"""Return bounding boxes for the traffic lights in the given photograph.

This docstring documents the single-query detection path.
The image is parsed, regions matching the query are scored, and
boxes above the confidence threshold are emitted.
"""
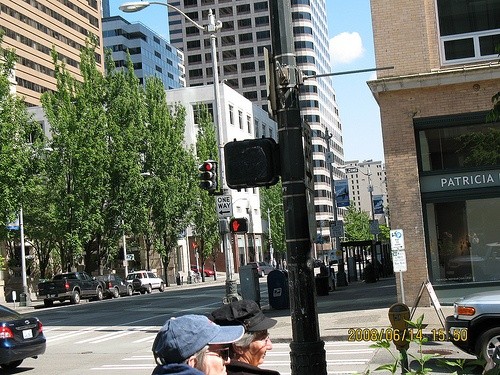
[205,161,218,191]
[199,165,205,189]
[230,218,248,234]
[223,136,279,195]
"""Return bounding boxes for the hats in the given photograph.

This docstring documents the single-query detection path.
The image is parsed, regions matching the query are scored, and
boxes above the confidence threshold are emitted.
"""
[208,299,277,331]
[152,315,244,364]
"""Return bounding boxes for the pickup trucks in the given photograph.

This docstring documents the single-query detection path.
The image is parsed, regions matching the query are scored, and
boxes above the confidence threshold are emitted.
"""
[38,270,104,307]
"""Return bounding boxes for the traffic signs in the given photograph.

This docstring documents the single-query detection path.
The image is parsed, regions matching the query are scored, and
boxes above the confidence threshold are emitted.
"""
[216,195,233,218]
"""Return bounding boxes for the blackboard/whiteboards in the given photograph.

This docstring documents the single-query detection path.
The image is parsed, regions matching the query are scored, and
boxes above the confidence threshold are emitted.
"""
[412,280,442,311]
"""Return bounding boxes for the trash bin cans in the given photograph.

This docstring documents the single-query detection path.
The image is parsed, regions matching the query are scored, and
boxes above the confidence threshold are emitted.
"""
[176,271,183,285]
[187,270,194,284]
[315,274,331,296]
[267,270,290,309]
[363,263,377,283]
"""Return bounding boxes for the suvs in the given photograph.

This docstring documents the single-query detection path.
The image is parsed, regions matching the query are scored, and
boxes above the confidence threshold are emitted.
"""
[248,261,274,277]
[126,270,165,294]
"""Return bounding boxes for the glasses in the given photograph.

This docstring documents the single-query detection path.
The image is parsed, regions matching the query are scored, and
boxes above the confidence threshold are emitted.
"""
[206,343,229,361]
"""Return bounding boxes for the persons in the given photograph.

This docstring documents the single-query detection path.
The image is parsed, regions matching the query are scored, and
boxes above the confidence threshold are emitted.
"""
[151,314,245,375]
[209,299,280,375]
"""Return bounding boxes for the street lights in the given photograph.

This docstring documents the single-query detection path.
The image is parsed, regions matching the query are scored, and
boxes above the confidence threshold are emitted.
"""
[337,165,378,242]
[118,1,244,307]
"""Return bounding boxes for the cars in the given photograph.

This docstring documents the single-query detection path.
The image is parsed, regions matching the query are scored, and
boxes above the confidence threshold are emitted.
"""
[445,289,500,370]
[0,303,47,371]
[191,265,215,277]
[93,274,134,298]
